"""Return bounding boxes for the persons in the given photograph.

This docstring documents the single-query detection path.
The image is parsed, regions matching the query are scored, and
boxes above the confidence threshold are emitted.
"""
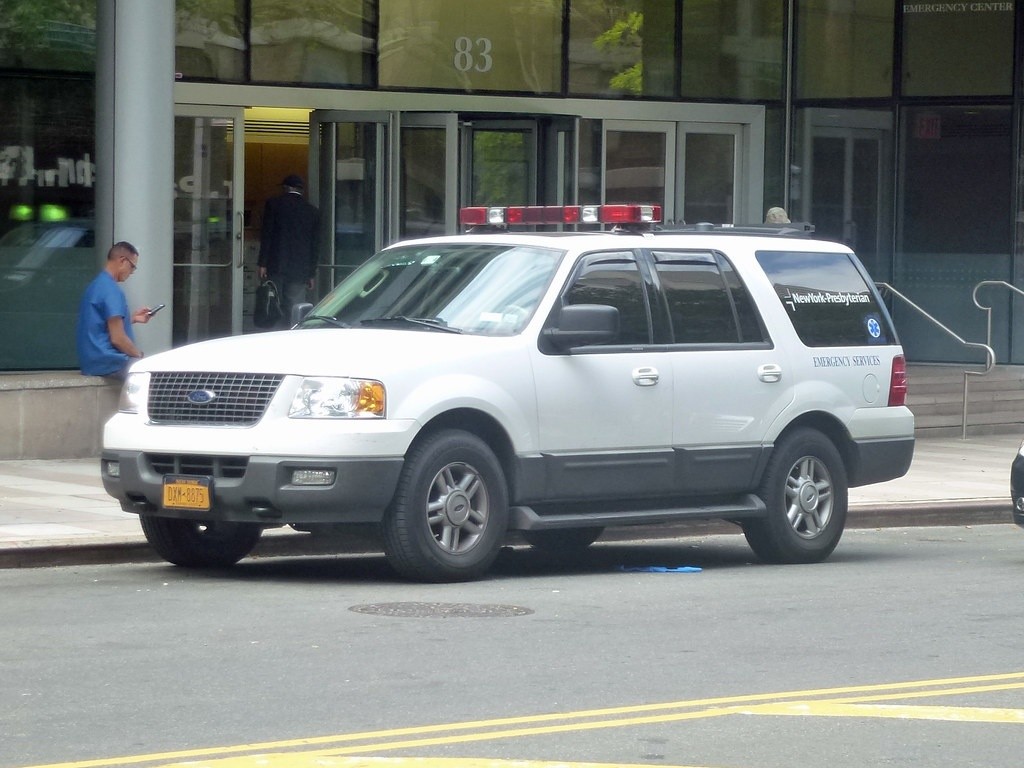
[257,173,325,334]
[76,241,154,380]
[763,206,790,226]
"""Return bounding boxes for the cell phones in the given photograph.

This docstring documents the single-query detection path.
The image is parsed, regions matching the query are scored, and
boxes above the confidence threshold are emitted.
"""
[147,304,165,315]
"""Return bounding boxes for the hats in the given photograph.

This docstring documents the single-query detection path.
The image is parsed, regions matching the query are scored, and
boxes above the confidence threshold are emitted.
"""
[276,174,305,189]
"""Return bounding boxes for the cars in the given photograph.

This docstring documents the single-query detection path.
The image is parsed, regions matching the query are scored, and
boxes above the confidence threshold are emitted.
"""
[2,220,229,368]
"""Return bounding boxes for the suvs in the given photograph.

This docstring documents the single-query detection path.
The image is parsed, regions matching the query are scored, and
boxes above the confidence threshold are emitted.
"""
[100,202,917,585]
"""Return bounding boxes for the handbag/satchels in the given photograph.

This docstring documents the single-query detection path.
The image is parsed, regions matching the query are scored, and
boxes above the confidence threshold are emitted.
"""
[253,271,286,328]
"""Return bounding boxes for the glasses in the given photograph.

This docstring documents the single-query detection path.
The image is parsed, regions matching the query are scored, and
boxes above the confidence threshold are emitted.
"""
[120,255,137,270]
